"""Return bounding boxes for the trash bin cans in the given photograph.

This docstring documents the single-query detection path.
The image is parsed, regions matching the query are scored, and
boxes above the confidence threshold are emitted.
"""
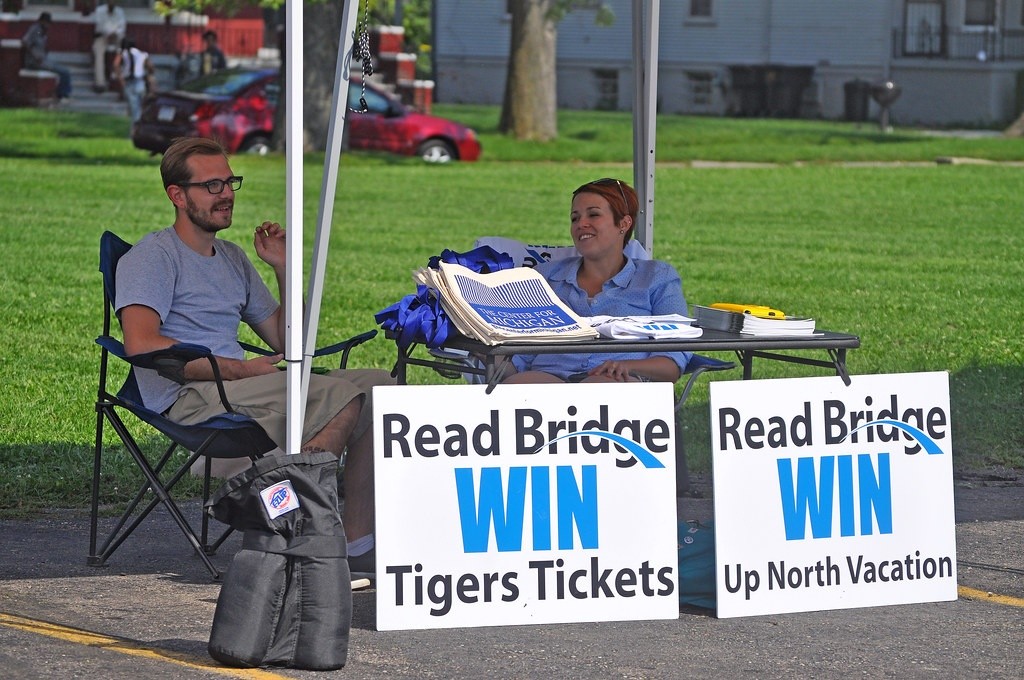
[728,63,814,118]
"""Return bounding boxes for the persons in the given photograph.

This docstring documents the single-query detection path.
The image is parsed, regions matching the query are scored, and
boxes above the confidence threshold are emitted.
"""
[113,135,399,579]
[138,56,160,108]
[21,11,55,106]
[472,177,694,384]
[197,30,227,78]
[112,37,154,140]
[91,0,127,95]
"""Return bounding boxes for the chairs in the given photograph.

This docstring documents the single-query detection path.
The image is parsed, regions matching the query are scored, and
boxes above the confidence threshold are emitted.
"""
[424,236,737,499]
[84,229,378,580]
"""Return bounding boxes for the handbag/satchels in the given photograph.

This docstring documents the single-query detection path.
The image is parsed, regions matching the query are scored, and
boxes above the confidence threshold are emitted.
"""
[372,239,601,355]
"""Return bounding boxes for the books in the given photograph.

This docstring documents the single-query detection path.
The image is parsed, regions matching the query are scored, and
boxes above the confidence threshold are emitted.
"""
[690,303,825,337]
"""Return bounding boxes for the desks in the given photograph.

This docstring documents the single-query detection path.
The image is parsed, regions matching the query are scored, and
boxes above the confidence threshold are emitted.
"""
[382,320,861,387]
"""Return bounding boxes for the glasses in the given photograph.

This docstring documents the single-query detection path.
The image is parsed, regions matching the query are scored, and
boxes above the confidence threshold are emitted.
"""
[572,177,630,215]
[174,175,243,194]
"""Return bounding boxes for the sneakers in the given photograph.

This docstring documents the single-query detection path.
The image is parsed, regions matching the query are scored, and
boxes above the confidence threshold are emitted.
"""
[347,547,376,580]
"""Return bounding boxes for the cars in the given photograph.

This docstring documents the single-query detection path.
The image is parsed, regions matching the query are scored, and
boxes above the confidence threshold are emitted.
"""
[133,68,483,168]
